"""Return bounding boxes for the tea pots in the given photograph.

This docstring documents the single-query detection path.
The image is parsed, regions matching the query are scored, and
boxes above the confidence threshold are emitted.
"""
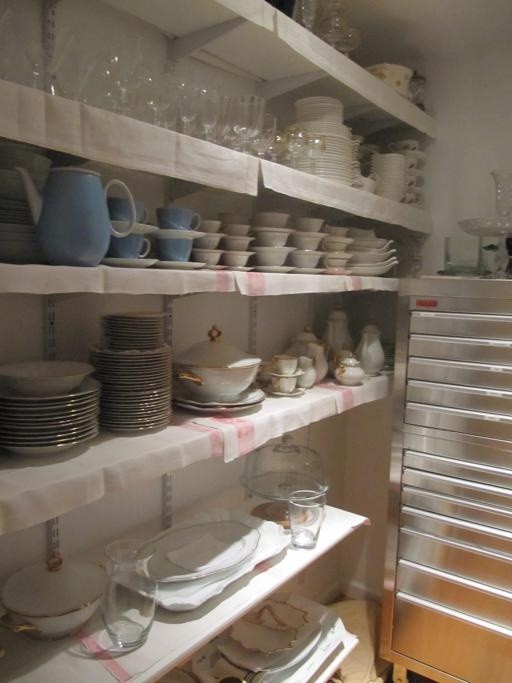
[12,160,138,270]
[294,304,388,388]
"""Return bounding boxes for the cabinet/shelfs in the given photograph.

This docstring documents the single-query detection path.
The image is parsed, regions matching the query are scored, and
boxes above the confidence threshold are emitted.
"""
[0,0,437,683]
[373,272,511,683]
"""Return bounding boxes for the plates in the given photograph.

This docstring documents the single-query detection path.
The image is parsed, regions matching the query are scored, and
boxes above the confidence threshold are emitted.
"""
[175,387,265,414]
[1,162,40,263]
[189,594,346,683]
[130,521,258,586]
[90,309,175,437]
[343,225,401,276]
[0,380,106,459]
[279,94,407,202]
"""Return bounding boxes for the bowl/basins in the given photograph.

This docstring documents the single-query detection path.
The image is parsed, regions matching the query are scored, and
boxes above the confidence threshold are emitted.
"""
[1,357,96,396]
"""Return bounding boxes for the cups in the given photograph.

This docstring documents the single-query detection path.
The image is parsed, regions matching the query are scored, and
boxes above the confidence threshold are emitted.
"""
[269,352,304,397]
[364,61,414,100]
[490,168,511,216]
[197,208,354,274]
[389,136,425,206]
[100,195,206,267]
[286,489,329,555]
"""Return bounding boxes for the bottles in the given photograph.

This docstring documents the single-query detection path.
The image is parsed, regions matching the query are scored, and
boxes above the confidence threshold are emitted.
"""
[101,536,156,651]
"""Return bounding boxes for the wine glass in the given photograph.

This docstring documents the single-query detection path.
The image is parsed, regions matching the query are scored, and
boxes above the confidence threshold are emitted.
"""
[11,14,329,173]
[455,215,512,280]
[298,0,361,58]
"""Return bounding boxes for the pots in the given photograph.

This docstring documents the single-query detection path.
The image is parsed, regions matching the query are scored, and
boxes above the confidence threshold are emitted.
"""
[2,553,107,643]
[174,323,262,402]
